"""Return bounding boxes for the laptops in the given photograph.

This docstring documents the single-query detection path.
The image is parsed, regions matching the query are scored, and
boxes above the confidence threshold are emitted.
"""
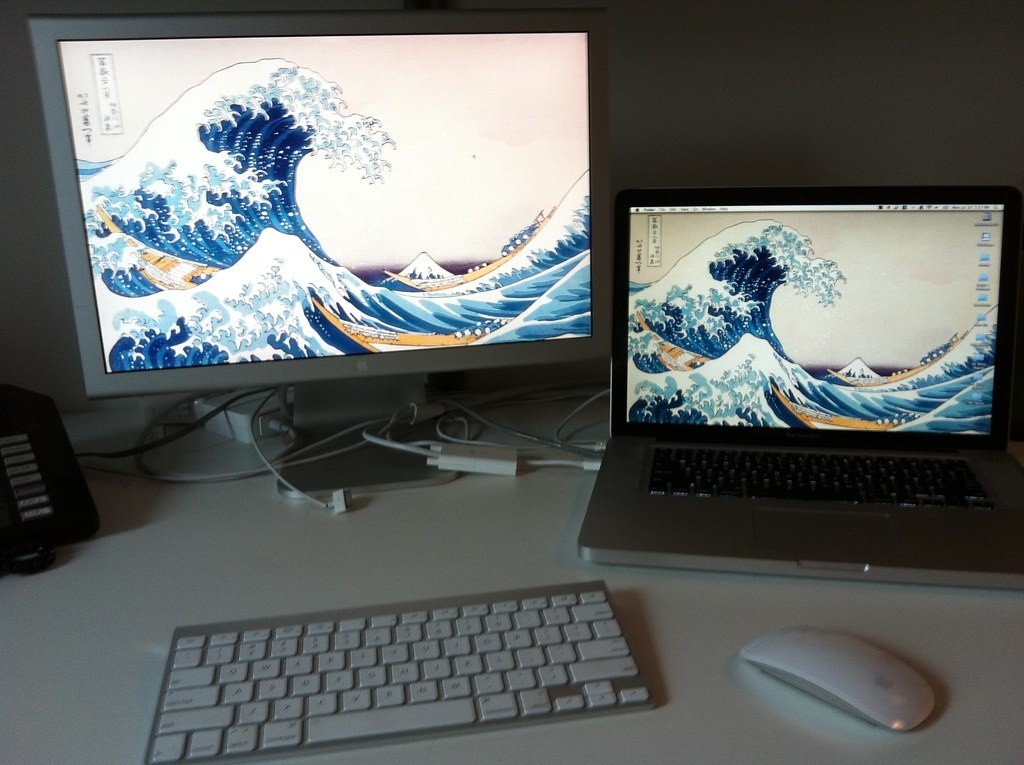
[577,187,1023,589]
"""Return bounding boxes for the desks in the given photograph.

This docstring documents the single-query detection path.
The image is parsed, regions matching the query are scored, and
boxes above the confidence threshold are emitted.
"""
[0,383,1021,762]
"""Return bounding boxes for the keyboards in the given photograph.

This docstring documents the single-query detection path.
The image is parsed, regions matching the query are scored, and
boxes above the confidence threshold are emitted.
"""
[144,580,656,765]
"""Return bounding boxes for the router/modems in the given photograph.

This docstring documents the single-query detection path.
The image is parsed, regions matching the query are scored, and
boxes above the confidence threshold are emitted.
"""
[192,391,295,443]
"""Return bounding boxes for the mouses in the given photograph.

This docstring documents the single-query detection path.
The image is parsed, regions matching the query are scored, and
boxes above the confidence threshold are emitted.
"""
[741,625,938,732]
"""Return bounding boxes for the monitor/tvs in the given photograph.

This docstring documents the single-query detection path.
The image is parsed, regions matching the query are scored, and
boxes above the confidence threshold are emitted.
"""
[23,13,613,497]
